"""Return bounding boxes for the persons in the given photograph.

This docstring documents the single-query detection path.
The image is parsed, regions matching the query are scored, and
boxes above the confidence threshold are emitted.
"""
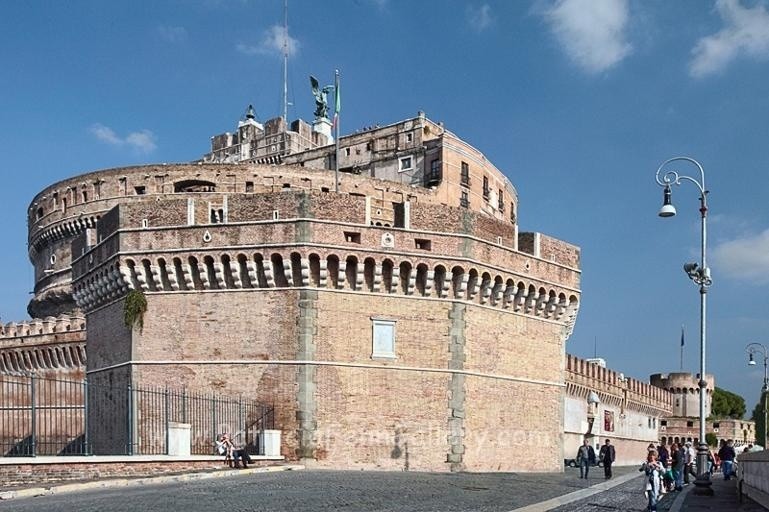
[599,438,616,479]
[576,439,595,479]
[222,433,256,468]
[320,87,328,118]
[639,438,754,511]
[215,433,244,468]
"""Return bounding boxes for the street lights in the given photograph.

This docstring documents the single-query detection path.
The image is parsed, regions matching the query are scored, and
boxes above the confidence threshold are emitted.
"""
[744,342,768,450]
[654,155,715,496]
